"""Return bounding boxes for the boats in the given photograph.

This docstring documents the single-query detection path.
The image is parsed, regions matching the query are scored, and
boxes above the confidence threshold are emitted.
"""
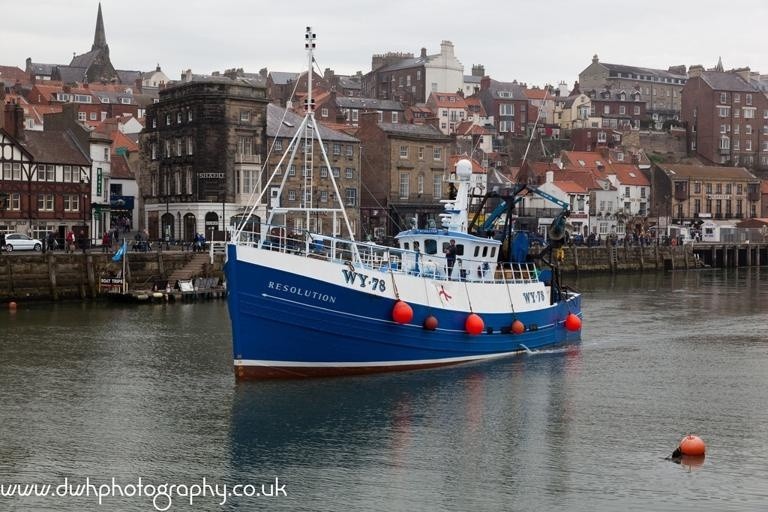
[224,21,593,382]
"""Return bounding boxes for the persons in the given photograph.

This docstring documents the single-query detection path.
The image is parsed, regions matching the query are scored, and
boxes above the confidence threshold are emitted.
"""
[194,233,201,252]
[46,217,151,253]
[200,234,206,251]
[694,231,699,243]
[443,239,456,281]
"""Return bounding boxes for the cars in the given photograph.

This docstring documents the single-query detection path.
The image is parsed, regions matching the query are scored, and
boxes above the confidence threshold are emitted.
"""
[2,233,48,253]
